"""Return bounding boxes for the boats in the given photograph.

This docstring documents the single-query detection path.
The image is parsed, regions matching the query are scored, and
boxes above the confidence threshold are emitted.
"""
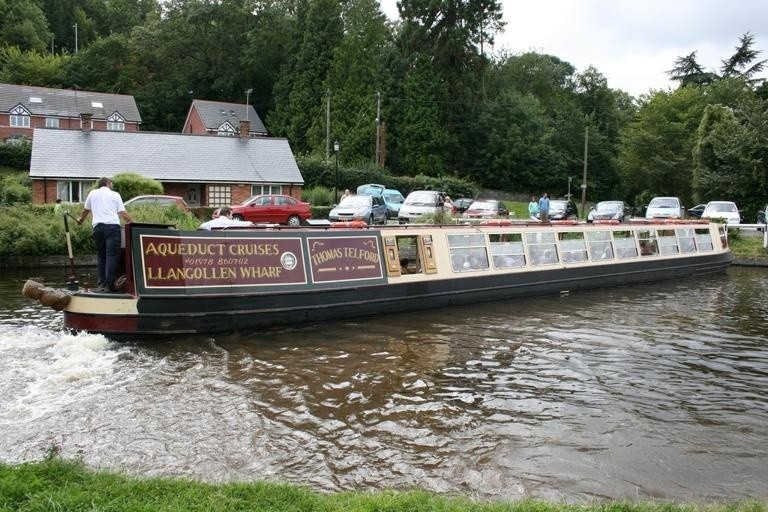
[50,202,735,340]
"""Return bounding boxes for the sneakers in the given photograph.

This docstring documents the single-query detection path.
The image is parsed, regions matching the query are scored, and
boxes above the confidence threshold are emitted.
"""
[104,283,119,293]
[98,282,105,291]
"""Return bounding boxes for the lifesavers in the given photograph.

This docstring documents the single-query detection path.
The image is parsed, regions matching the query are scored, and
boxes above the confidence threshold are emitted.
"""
[550,218,581,226]
[480,218,511,227]
[594,219,620,226]
[331,220,367,231]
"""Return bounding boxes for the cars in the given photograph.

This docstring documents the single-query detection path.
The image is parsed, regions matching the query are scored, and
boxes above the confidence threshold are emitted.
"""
[686,204,707,219]
[586,201,626,223]
[399,190,447,224]
[209,190,313,228]
[324,183,391,228]
[462,200,509,222]
[756,206,767,230]
[452,197,474,213]
[701,202,741,230]
[645,197,685,221]
[379,189,407,216]
[536,201,581,224]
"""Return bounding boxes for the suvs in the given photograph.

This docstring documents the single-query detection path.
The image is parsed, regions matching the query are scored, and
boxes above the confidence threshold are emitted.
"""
[118,195,197,223]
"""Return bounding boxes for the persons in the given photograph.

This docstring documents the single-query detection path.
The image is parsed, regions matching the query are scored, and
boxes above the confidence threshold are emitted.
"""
[444,195,456,218]
[400,258,411,274]
[452,241,654,270]
[537,191,550,221]
[340,189,351,202]
[529,195,539,220]
[52,196,64,216]
[198,205,255,231]
[77,177,137,293]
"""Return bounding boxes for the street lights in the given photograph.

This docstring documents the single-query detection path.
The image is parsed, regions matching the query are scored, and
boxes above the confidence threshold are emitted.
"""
[333,137,340,204]
